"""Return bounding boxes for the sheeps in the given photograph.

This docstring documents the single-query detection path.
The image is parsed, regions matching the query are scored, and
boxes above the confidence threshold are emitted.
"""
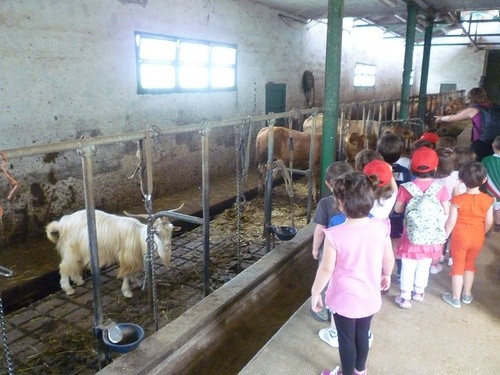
[45,202,184,299]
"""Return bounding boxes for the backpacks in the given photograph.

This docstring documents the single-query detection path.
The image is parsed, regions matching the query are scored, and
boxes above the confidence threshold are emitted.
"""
[399,180,447,245]
[471,104,500,144]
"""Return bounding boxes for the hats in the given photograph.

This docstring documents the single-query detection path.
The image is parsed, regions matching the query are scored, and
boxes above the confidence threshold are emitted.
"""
[412,146,439,172]
[420,131,441,143]
[363,160,392,187]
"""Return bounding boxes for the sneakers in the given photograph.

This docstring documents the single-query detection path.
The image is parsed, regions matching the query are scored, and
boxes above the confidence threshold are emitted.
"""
[321,366,342,375]
[461,293,473,304]
[368,334,374,348]
[318,327,339,347]
[354,366,367,375]
[395,295,411,309]
[442,293,461,308]
[412,290,423,301]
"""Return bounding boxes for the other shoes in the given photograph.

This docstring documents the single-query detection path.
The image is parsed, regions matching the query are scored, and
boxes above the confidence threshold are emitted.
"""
[396,273,401,281]
[430,264,442,273]
[439,255,445,262]
[311,305,330,321]
[447,257,453,266]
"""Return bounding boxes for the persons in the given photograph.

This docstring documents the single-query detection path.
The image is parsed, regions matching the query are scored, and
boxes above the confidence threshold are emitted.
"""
[434,87,500,142]
[394,146,450,309]
[354,128,496,295]
[442,161,494,308]
[310,161,355,322]
[311,171,395,375]
[481,135,500,226]
[319,213,375,348]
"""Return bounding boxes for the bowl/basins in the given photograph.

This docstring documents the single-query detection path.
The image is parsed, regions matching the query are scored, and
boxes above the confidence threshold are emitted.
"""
[275,227,297,240]
[101,322,144,353]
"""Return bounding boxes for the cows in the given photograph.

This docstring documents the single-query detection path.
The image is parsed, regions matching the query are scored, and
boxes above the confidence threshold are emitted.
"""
[255,94,467,205]
